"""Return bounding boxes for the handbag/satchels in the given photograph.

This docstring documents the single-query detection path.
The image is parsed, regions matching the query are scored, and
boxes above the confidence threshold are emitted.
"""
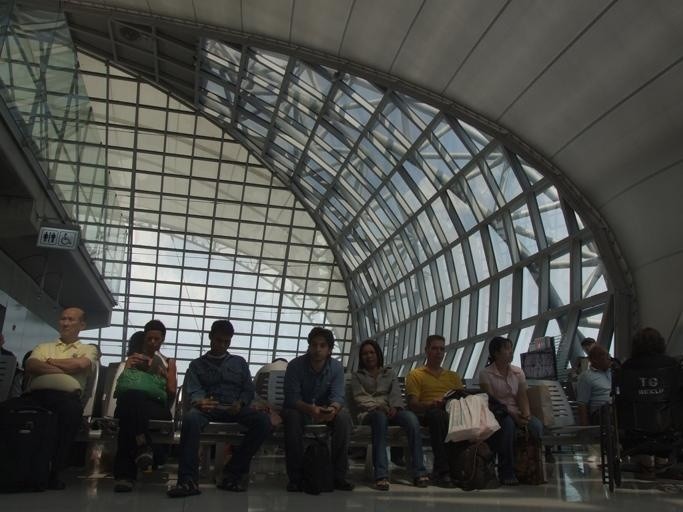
[452,440,499,492]
[513,426,542,486]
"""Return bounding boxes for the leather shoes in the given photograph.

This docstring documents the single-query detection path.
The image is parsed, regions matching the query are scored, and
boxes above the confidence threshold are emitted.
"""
[50,473,65,490]
[286,479,303,492]
[390,456,407,467]
[335,477,355,490]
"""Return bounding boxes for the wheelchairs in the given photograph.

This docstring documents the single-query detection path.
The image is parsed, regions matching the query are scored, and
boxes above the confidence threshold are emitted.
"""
[600,353,683,493]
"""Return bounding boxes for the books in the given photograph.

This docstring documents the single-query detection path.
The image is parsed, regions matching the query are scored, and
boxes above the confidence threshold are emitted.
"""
[146,350,169,376]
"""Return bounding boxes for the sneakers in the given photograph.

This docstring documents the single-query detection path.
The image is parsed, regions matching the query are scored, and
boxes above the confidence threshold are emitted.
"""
[134,446,153,468]
[113,475,134,491]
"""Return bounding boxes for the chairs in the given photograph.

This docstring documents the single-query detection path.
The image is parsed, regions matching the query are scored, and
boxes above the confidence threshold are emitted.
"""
[0,354,600,489]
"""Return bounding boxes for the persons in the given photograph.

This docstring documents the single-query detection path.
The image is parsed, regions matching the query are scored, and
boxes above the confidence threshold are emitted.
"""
[403,333,499,487]
[579,335,622,386]
[22,306,99,490]
[112,318,177,493]
[349,338,431,490]
[163,319,272,498]
[279,326,356,491]
[126,330,144,354]
[0,303,7,349]
[613,325,682,473]
[477,335,548,485]
[574,344,669,473]
[86,341,105,416]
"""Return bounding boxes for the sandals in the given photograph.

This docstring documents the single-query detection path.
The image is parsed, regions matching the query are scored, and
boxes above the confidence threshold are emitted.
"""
[376,478,389,491]
[168,482,202,496]
[216,476,244,491]
[414,475,430,488]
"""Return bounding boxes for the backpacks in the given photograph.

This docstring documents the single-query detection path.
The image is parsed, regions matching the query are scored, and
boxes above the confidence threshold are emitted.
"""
[306,431,334,496]
[1,398,58,493]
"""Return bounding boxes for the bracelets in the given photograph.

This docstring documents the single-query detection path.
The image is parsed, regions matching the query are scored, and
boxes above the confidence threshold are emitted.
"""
[329,404,337,411]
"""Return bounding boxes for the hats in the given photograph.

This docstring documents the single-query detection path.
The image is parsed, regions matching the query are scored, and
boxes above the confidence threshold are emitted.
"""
[581,338,594,346]
